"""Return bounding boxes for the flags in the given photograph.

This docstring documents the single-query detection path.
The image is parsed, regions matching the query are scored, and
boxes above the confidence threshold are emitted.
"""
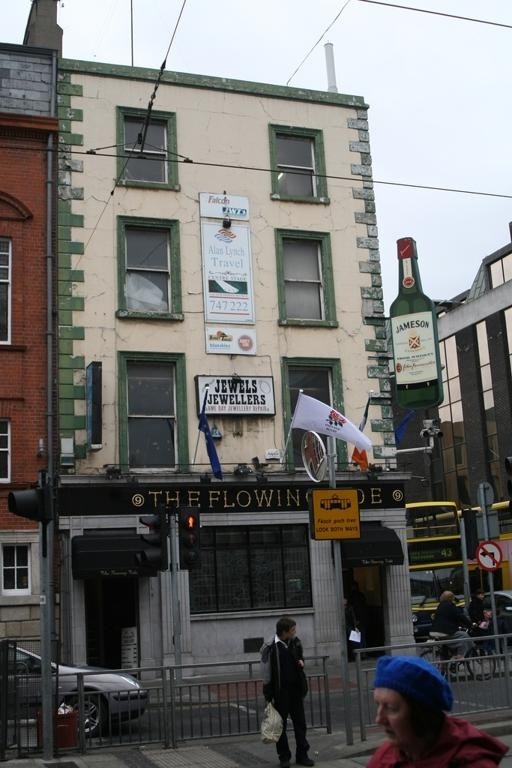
[393,408,414,440]
[197,391,223,480]
[291,393,371,450]
[352,395,371,473]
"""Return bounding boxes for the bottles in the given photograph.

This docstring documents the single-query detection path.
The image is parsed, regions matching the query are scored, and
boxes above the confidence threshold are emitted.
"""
[387,235,444,410]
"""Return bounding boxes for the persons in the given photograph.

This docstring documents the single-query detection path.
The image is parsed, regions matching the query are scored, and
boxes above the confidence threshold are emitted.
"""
[257,618,315,767]
[364,655,509,768]
[465,592,494,655]
[431,588,481,678]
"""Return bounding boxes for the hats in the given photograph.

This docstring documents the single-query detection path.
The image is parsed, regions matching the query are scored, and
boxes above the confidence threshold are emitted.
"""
[375,654,453,717]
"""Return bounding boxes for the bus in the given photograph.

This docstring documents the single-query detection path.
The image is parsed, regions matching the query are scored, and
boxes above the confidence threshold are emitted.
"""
[404,501,511,649]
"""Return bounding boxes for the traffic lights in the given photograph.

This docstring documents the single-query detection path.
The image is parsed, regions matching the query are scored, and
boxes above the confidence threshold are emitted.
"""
[178,505,203,570]
[138,503,169,569]
[503,456,512,507]
[8,483,52,526]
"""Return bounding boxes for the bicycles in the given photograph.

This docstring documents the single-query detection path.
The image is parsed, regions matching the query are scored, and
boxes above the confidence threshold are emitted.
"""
[419,625,496,681]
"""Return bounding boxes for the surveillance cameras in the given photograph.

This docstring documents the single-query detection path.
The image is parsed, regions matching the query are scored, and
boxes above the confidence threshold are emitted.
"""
[419,418,443,440]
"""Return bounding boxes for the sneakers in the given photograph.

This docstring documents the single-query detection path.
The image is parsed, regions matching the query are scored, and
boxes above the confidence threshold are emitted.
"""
[281,760,293,767]
[296,757,314,766]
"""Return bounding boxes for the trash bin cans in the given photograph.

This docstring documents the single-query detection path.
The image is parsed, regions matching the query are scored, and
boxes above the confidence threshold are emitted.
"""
[36,708,79,749]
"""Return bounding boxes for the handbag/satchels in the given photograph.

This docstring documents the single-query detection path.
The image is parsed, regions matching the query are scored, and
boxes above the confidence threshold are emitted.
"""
[260,702,284,744]
[298,666,308,700]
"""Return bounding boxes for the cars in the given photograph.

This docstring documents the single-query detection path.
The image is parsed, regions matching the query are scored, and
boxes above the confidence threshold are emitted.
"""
[1,641,151,741]
[263,568,306,605]
[190,577,242,613]
